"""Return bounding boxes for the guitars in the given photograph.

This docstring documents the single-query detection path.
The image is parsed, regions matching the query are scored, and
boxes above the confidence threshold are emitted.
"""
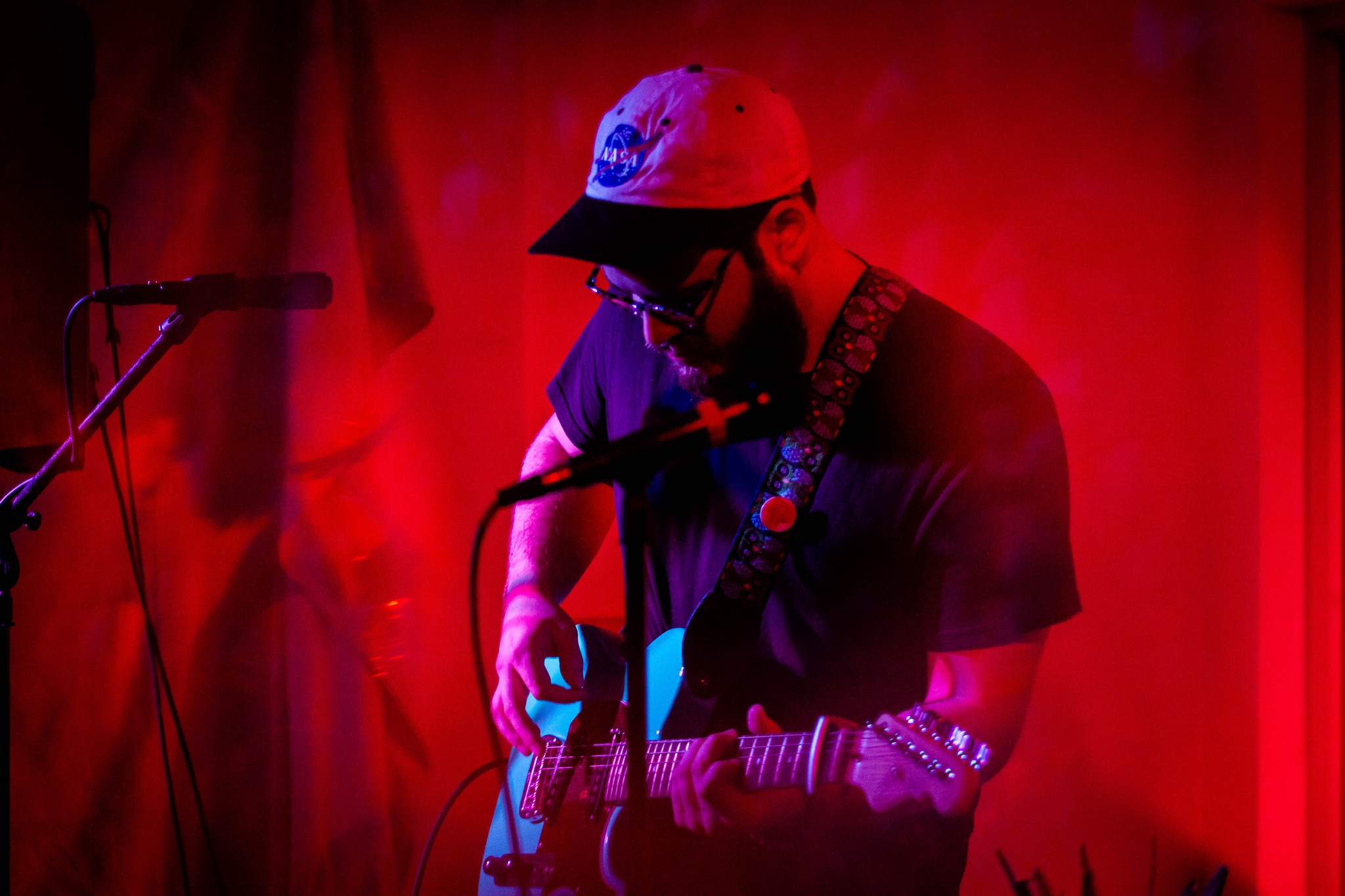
[475,623,1000,895]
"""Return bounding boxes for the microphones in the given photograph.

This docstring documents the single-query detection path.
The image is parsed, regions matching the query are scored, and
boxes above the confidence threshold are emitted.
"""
[497,381,772,511]
[98,270,332,311]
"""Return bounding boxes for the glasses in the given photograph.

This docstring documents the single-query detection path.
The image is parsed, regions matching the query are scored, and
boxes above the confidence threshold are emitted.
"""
[578,242,745,338]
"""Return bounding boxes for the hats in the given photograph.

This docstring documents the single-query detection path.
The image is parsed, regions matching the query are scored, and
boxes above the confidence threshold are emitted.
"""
[527,63,817,290]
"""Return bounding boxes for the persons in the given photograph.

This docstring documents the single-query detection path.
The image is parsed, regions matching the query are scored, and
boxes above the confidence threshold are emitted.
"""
[488,67,1082,896]
